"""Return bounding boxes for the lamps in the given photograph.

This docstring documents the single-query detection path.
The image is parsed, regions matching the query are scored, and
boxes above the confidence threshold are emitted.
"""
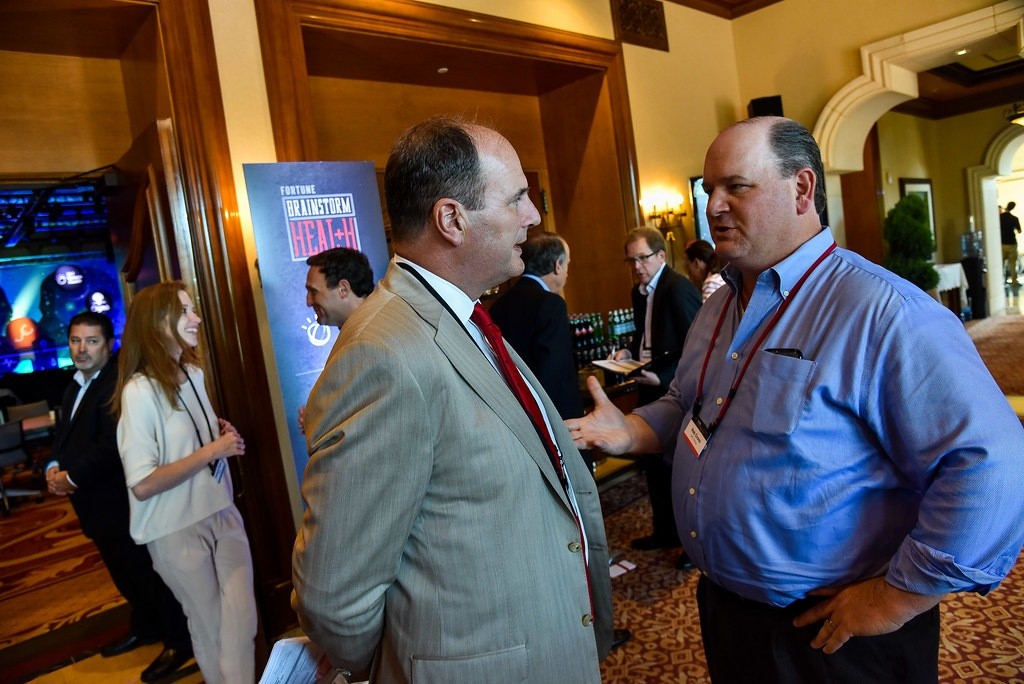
[639,185,689,244]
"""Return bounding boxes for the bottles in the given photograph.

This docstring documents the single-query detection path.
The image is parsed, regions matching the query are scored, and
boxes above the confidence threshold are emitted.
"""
[961,231,984,259]
[570,308,634,372]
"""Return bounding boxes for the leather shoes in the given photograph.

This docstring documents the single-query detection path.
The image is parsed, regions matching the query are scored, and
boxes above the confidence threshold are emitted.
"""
[612,628,631,647]
[100,632,156,657]
[140,638,194,683]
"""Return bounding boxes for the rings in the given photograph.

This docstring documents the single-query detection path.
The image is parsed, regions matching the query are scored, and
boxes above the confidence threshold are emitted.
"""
[828,618,837,632]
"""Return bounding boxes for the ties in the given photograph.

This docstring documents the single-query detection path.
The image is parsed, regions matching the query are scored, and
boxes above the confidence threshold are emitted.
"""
[473,299,566,493]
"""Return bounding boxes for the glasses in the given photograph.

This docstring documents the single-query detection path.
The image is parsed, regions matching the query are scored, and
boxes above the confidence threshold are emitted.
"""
[625,248,660,265]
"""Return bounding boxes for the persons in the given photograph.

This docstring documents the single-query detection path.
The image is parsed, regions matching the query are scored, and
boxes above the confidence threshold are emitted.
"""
[685,240,728,305]
[44,312,195,682]
[485,231,630,649]
[998,202,1024,287]
[99,279,256,684]
[287,112,616,684]
[298,247,375,435]
[563,117,1024,684]
[608,225,702,570]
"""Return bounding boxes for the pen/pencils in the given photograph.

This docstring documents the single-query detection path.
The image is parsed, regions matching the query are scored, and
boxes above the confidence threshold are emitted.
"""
[611,345,616,360]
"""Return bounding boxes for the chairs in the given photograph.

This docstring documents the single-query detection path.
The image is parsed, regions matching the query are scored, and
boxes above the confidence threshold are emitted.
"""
[0,404,75,516]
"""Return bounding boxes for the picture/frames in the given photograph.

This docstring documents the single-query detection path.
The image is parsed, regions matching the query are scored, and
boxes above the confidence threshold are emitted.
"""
[899,176,937,250]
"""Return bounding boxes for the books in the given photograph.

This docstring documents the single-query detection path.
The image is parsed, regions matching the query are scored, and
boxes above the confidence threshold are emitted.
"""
[592,357,651,379]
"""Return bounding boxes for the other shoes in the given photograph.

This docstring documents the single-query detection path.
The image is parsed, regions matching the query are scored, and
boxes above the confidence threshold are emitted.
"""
[675,553,696,571]
[631,531,683,551]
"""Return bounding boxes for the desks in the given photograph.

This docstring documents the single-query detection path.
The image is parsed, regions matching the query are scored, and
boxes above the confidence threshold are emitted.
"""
[24,413,56,432]
[928,263,969,310]
